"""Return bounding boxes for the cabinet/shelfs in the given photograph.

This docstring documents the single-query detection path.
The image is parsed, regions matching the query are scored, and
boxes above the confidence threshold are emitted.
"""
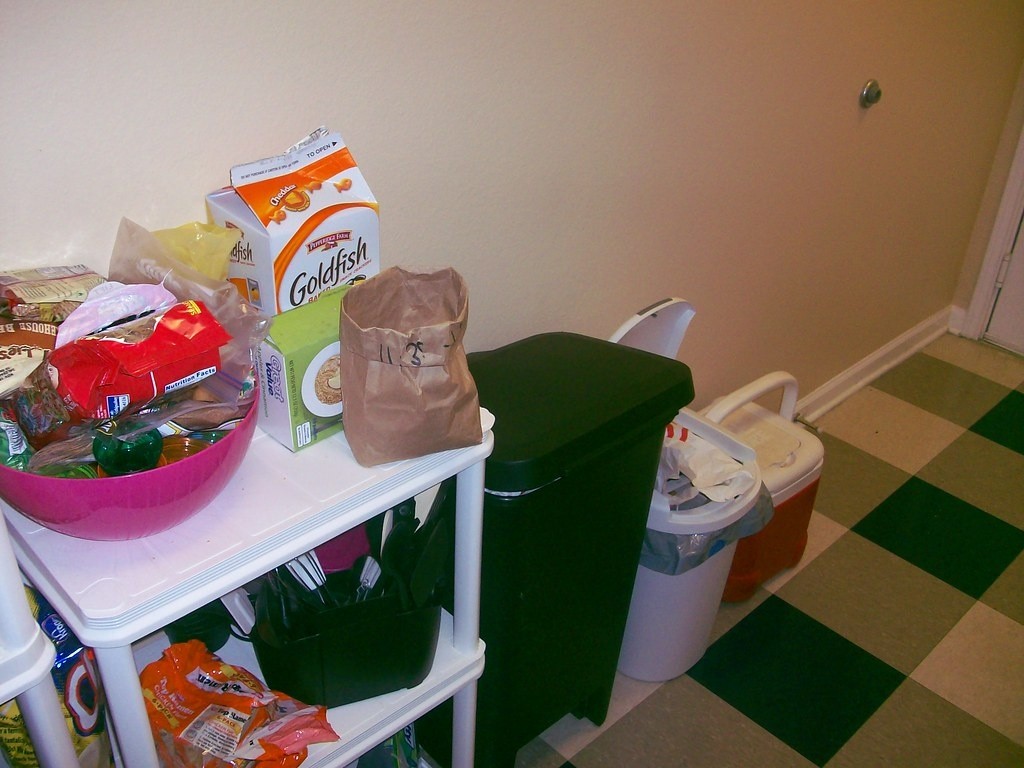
[0,406,494,768]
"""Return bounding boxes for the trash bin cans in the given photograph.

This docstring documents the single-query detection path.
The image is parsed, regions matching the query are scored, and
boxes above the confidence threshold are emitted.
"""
[607,294,765,682]
[410,328,698,766]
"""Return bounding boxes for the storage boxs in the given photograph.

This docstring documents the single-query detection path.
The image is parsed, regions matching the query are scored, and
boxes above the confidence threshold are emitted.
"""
[256,286,353,453]
[697,371,823,602]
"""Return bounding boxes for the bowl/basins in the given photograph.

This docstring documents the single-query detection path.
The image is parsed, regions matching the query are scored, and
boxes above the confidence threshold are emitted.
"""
[0,368,261,542]
